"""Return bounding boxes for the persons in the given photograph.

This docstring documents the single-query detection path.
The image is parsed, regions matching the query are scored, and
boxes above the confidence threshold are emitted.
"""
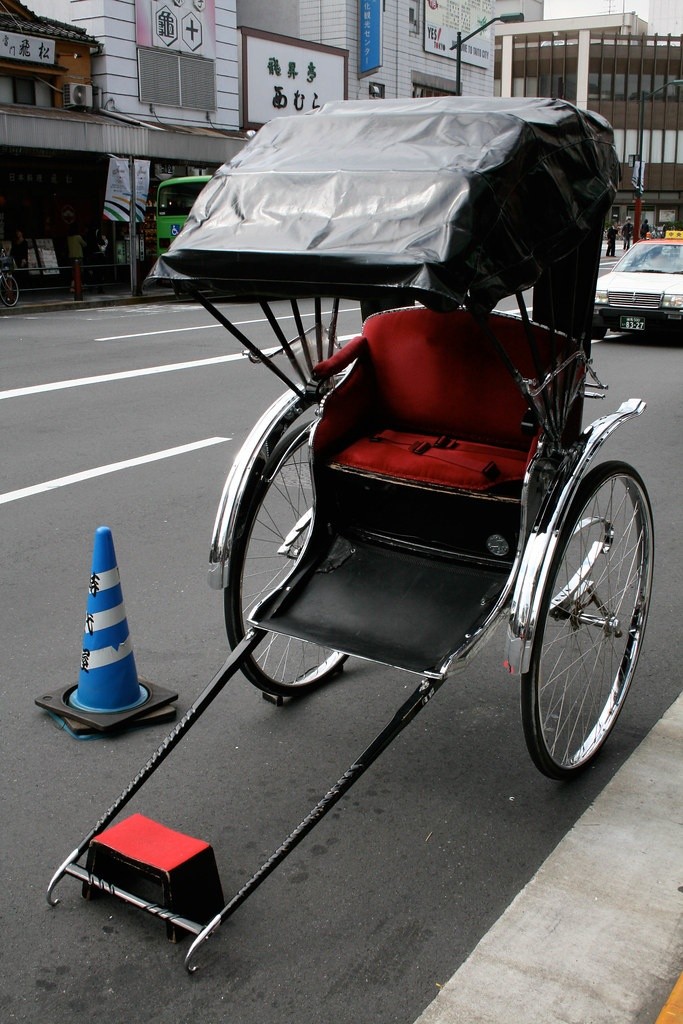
[621,219,633,251]
[606,222,618,257]
[9,231,29,269]
[641,219,649,237]
[66,226,87,291]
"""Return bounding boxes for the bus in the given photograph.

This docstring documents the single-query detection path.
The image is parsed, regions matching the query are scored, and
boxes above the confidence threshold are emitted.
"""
[150,175,215,259]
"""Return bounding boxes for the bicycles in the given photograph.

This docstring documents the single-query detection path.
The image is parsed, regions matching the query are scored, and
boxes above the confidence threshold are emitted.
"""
[0,249,19,307]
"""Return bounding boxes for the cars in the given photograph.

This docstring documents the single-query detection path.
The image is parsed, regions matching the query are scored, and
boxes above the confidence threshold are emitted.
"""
[591,230,683,345]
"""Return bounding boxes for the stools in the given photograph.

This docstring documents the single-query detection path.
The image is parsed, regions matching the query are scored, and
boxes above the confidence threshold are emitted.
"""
[81,813,226,942]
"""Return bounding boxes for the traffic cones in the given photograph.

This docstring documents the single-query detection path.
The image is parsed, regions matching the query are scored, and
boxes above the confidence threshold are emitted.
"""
[35,527,180,739]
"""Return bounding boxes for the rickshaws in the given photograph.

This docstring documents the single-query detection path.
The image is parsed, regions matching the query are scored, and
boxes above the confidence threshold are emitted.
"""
[44,95,658,977]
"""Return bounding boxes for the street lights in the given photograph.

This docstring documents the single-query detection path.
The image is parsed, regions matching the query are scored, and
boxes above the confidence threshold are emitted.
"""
[449,12,524,96]
[633,80,683,245]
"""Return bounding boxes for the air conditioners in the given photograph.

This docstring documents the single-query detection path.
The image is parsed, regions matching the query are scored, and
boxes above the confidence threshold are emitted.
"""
[63,82,93,108]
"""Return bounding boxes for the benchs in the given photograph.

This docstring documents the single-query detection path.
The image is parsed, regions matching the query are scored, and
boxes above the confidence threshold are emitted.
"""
[312,306,586,502]
